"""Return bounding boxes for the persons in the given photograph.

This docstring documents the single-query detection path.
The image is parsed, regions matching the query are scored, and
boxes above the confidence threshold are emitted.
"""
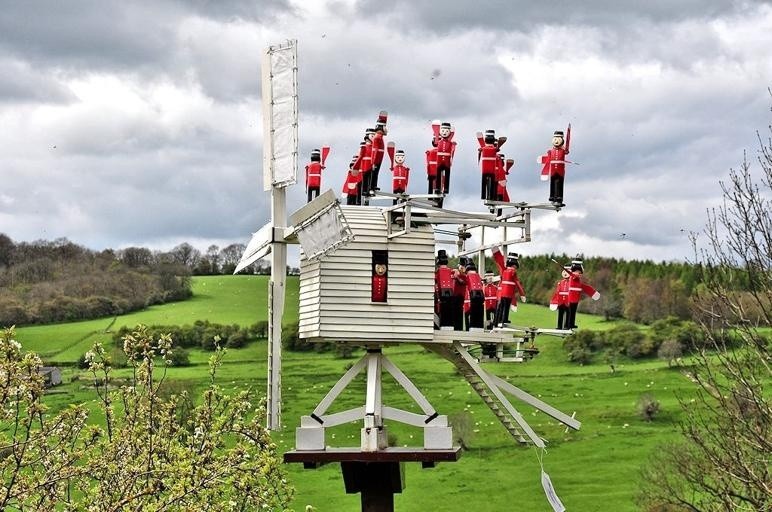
[306,147,330,203]
[434,247,527,331]
[372,259,388,302]
[425,120,457,209]
[476,129,514,222]
[537,123,571,208]
[305,111,599,334]
[343,112,410,207]
[549,257,599,330]
